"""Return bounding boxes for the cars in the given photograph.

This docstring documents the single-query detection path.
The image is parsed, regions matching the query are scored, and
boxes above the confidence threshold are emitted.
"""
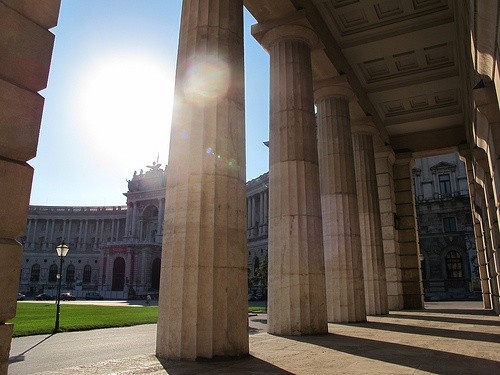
[60,293,76,301]
[85,291,104,300]
[17,293,26,301]
[34,293,55,300]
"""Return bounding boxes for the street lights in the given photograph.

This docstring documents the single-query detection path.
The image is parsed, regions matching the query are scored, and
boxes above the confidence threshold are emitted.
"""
[52,240,71,333]
[55,273,63,303]
